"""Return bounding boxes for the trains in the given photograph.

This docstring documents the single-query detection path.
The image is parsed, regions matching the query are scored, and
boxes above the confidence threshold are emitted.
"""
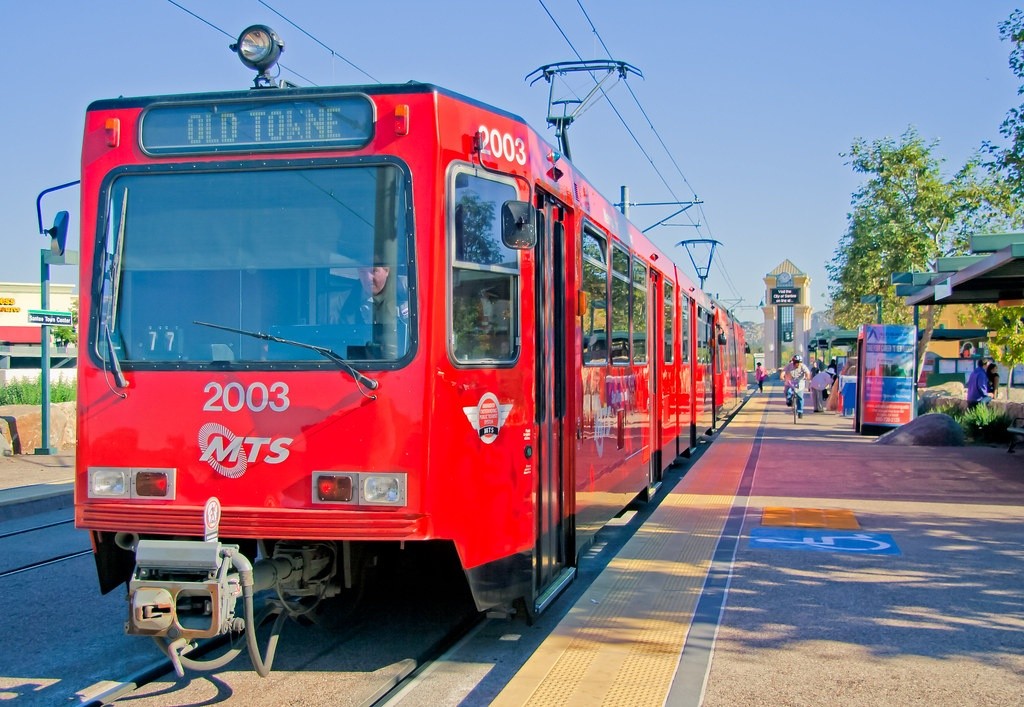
[37,23,750,677]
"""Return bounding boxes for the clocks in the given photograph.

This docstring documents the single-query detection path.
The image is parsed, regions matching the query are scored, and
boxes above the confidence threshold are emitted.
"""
[779,272,791,284]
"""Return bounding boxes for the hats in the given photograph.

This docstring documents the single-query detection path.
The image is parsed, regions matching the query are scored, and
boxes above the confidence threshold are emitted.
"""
[826,368,836,375]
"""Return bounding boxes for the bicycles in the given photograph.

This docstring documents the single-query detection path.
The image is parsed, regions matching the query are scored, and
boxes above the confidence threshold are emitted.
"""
[780,379,807,425]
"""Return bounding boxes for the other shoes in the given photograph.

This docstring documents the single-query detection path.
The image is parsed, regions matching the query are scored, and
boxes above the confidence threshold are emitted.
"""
[798,413,802,418]
[786,400,792,406]
[814,409,824,413]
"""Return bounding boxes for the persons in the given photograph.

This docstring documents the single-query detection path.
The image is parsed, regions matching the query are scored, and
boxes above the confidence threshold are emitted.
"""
[779,355,811,419]
[811,368,836,412]
[341,252,408,361]
[810,362,819,378]
[756,362,767,393]
[966,357,999,410]
[828,359,837,387]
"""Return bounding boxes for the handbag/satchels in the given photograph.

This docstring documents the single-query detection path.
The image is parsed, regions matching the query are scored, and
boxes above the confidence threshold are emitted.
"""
[822,389,829,401]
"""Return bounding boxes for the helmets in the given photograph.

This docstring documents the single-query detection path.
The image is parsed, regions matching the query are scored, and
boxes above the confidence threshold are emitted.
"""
[792,355,802,362]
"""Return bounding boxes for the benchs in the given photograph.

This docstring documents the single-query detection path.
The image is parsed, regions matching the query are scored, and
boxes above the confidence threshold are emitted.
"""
[1007,417,1024,452]
